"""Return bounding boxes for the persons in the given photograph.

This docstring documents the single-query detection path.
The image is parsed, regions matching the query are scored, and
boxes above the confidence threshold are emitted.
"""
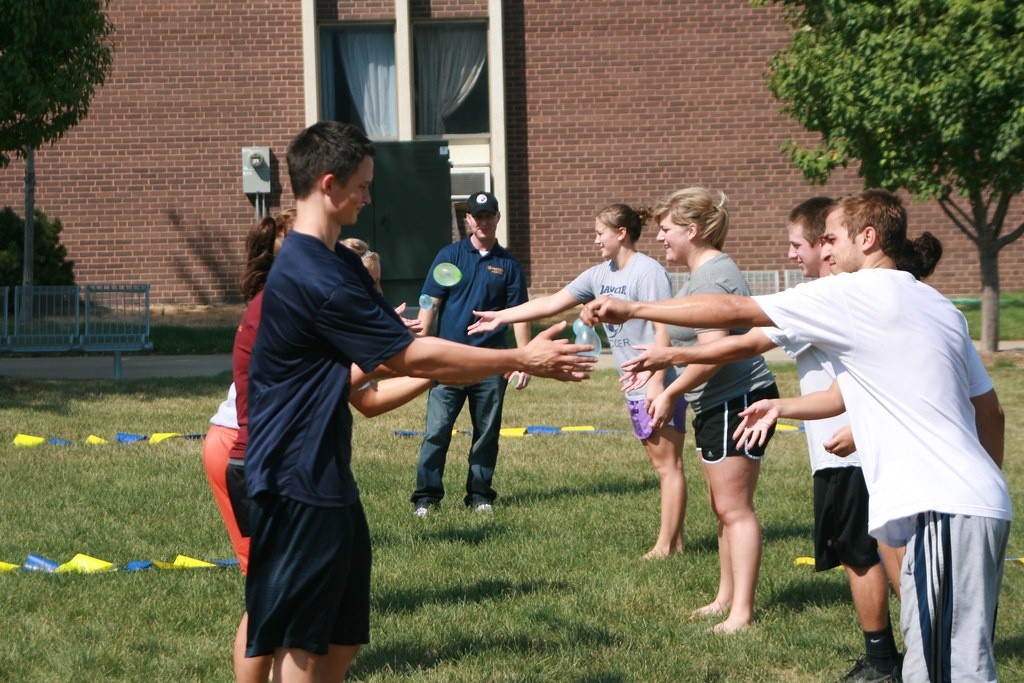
[620,197,907,683]
[242,118,600,683]
[202,237,429,577]
[224,209,298,682]
[617,188,781,634]
[466,202,688,559]
[410,192,532,518]
[581,190,1010,683]
[731,231,943,599]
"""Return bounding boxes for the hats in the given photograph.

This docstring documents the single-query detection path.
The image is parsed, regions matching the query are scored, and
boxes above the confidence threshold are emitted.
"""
[466,190,498,217]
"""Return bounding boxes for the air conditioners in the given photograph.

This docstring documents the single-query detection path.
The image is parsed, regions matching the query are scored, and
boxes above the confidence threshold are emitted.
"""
[436,167,490,201]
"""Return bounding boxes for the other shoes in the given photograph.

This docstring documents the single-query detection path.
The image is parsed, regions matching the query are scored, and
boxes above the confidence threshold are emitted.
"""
[471,502,493,513]
[412,501,428,517]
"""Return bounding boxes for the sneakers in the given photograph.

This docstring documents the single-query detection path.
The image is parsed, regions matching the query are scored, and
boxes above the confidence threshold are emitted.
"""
[836,652,905,683]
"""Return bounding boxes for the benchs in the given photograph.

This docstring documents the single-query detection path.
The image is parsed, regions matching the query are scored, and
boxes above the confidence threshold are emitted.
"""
[1,283,157,378]
[659,268,818,294]
